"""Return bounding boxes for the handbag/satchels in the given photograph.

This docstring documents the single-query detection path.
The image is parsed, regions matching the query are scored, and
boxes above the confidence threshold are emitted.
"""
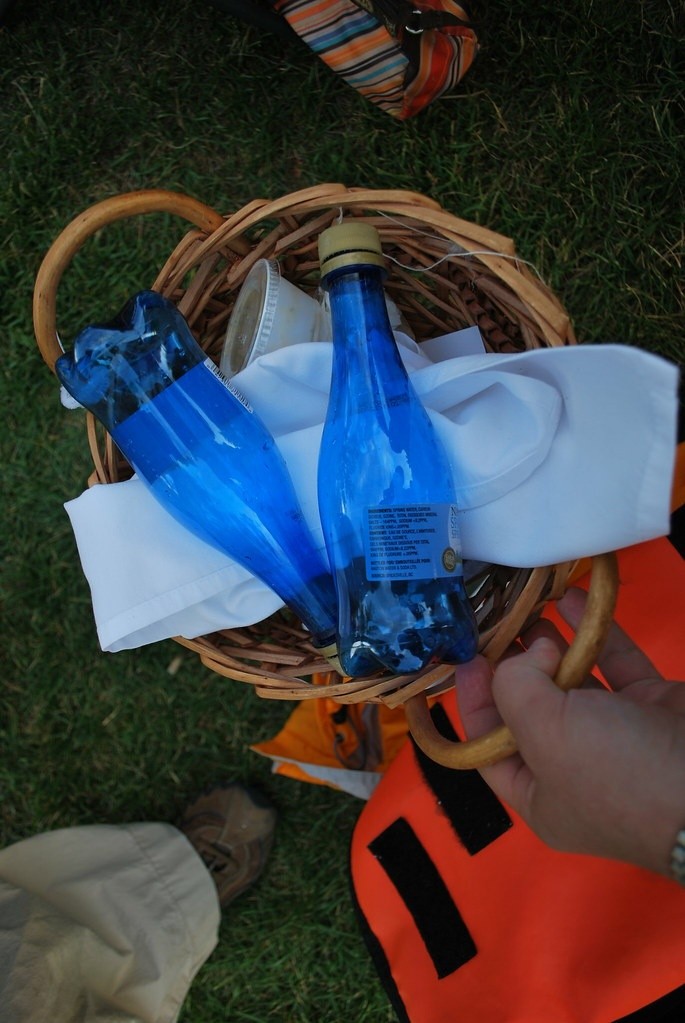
[273,0,481,121]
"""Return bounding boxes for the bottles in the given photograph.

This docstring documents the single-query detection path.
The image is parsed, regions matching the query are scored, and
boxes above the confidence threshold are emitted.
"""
[50,287,355,679]
[311,221,480,679]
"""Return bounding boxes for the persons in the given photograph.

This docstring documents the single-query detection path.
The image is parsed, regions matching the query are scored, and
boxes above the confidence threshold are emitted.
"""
[452,581,685,887]
[1,767,281,1022]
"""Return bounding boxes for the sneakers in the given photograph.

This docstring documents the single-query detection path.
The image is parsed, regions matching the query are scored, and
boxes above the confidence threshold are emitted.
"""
[173,780,279,909]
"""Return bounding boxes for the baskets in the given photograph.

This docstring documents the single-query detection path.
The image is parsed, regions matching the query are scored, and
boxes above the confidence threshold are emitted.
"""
[31,179,618,767]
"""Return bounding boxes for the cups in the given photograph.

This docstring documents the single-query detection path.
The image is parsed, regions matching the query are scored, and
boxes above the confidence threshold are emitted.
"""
[220,255,320,384]
